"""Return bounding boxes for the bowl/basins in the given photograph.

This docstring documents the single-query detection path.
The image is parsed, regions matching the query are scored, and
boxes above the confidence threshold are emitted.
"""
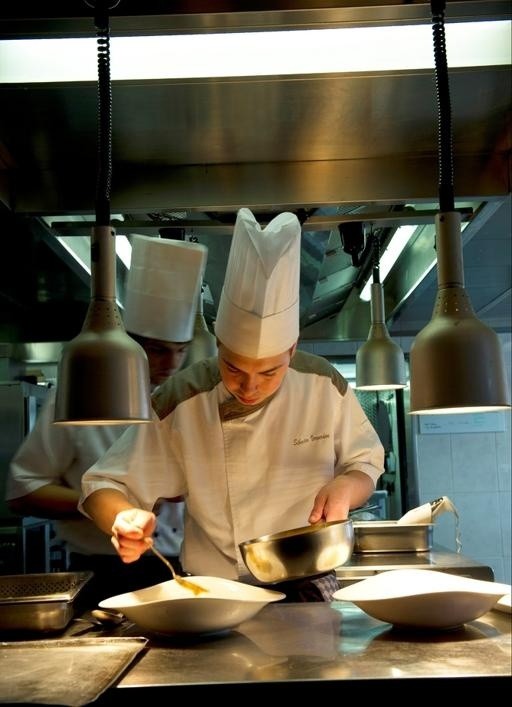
[97,574,287,640]
[330,568,511,633]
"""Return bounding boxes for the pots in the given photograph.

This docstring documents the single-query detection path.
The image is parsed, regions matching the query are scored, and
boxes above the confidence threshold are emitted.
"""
[237,517,356,587]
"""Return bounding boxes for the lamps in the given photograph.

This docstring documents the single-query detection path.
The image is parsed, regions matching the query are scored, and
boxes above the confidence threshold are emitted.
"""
[54,0,152,426]
[158,228,218,374]
[410,0,511,416]
[106,213,132,270]
[338,221,407,390]
[358,224,419,302]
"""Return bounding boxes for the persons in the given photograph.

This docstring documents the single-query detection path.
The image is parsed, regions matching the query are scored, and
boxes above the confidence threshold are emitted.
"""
[75,330,387,604]
[3,327,197,617]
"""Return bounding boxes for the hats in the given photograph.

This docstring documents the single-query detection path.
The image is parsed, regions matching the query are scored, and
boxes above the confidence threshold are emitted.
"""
[123,233,207,343]
[214,208,302,359]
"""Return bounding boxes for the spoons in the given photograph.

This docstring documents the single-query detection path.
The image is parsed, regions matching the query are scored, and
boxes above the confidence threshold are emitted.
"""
[148,543,209,595]
[91,608,125,626]
